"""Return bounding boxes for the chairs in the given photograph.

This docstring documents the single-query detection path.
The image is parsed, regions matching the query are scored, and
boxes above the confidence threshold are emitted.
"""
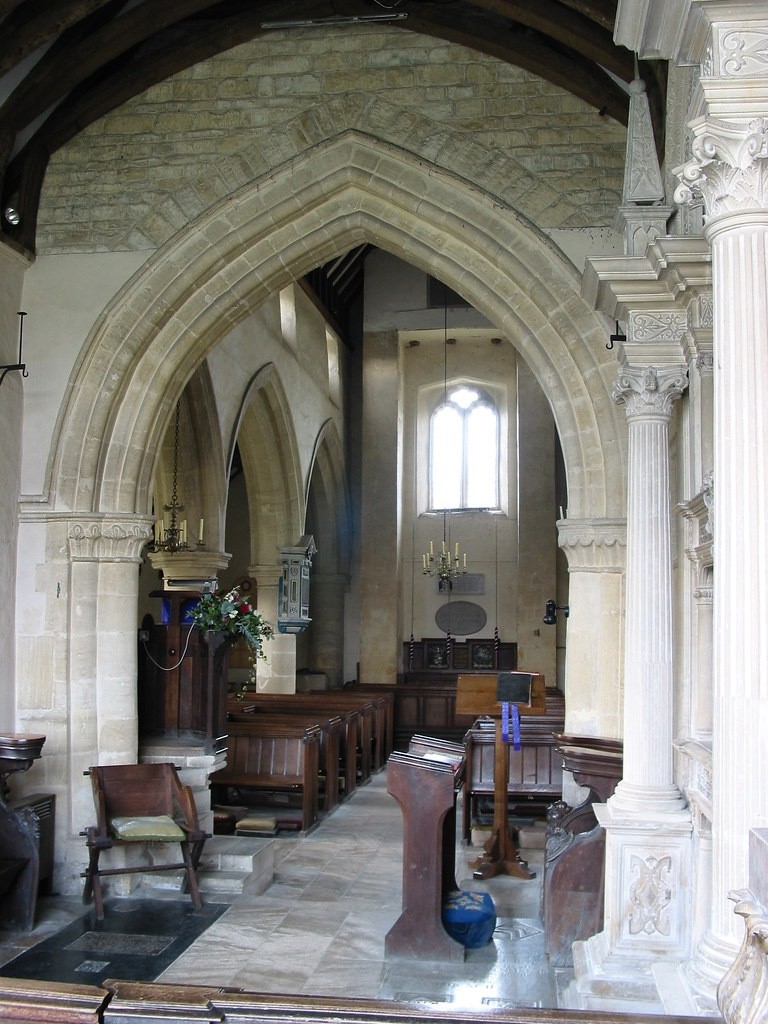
[79,762,213,922]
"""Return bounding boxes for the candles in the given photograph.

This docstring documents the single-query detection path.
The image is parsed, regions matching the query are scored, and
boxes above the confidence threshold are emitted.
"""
[422,539,466,578]
[154,518,205,547]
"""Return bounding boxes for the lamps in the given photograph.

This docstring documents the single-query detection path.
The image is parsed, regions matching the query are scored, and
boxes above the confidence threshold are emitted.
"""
[543,599,569,625]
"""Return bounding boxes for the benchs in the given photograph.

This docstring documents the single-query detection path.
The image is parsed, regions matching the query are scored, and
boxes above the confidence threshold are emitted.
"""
[226,692,398,840]
[459,686,567,846]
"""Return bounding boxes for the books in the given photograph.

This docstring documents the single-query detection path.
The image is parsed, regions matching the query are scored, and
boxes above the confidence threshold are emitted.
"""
[424,749,465,768]
[497,671,531,706]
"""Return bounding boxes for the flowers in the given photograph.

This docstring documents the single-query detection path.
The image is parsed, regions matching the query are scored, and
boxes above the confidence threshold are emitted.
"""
[188,582,272,703]
